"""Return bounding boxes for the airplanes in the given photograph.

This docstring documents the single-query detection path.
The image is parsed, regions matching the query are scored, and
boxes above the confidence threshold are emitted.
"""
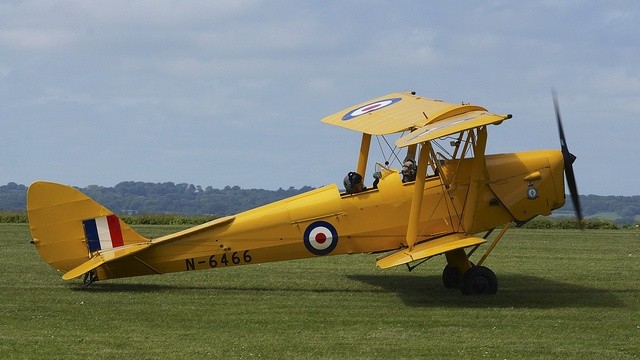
[28,91,586,298]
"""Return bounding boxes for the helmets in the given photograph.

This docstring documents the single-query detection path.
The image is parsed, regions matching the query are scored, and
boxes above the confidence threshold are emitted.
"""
[343,172,362,192]
[402,159,416,176]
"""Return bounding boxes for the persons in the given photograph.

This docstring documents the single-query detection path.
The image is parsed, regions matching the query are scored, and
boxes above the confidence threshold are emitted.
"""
[401,160,419,184]
[344,172,366,194]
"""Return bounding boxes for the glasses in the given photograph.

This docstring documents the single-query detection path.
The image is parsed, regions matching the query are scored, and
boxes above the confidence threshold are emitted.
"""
[348,172,355,188]
